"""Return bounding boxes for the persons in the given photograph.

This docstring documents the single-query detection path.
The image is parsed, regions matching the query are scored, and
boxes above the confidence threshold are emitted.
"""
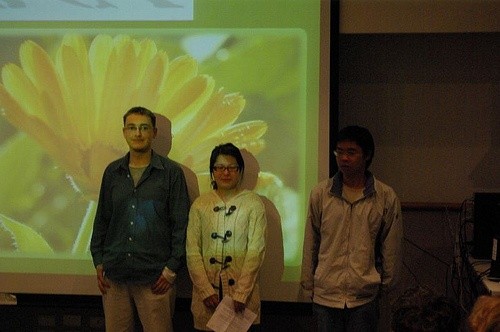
[90,106,191,332]
[185,143,267,332]
[302,126,403,332]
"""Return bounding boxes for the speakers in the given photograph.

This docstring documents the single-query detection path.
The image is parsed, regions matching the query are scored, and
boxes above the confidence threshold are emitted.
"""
[469,192,500,259]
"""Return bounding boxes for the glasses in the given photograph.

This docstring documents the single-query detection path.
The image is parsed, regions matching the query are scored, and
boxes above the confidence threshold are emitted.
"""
[124,126,153,131]
[212,165,239,172]
[334,151,363,157]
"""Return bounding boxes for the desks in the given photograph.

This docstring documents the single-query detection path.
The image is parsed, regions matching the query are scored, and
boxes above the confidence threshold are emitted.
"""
[466,254,500,298]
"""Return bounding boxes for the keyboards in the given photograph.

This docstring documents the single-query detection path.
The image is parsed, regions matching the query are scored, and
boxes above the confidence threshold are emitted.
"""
[488,260,500,281]
